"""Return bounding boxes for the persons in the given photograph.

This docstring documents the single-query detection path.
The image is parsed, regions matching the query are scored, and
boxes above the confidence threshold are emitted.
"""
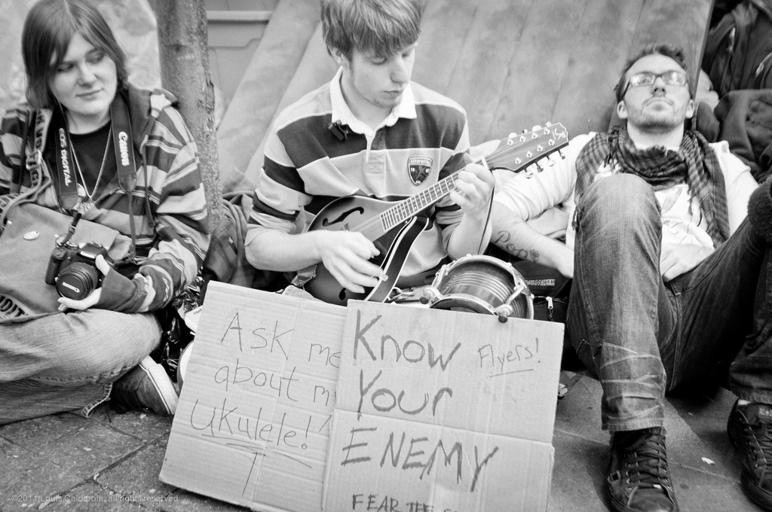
[241,2,501,310]
[488,39,771,509]
[1,0,216,436]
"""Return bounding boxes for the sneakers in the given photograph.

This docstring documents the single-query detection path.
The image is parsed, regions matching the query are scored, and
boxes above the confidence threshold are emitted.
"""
[108,356,181,416]
[603,427,678,512]
[729,399,771,511]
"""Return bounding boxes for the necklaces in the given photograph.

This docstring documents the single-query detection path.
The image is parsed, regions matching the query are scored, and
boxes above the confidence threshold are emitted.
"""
[68,127,115,208]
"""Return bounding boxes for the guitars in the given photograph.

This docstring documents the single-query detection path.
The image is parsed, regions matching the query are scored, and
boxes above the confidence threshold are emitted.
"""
[295,121,570,307]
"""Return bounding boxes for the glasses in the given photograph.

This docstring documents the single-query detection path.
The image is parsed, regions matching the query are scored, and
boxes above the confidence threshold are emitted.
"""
[620,70,693,100]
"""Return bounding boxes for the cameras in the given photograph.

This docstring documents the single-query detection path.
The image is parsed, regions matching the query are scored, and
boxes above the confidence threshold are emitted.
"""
[45,240,116,301]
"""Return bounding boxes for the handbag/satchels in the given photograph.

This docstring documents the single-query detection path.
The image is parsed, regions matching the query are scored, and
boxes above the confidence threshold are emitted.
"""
[4,109,131,320]
